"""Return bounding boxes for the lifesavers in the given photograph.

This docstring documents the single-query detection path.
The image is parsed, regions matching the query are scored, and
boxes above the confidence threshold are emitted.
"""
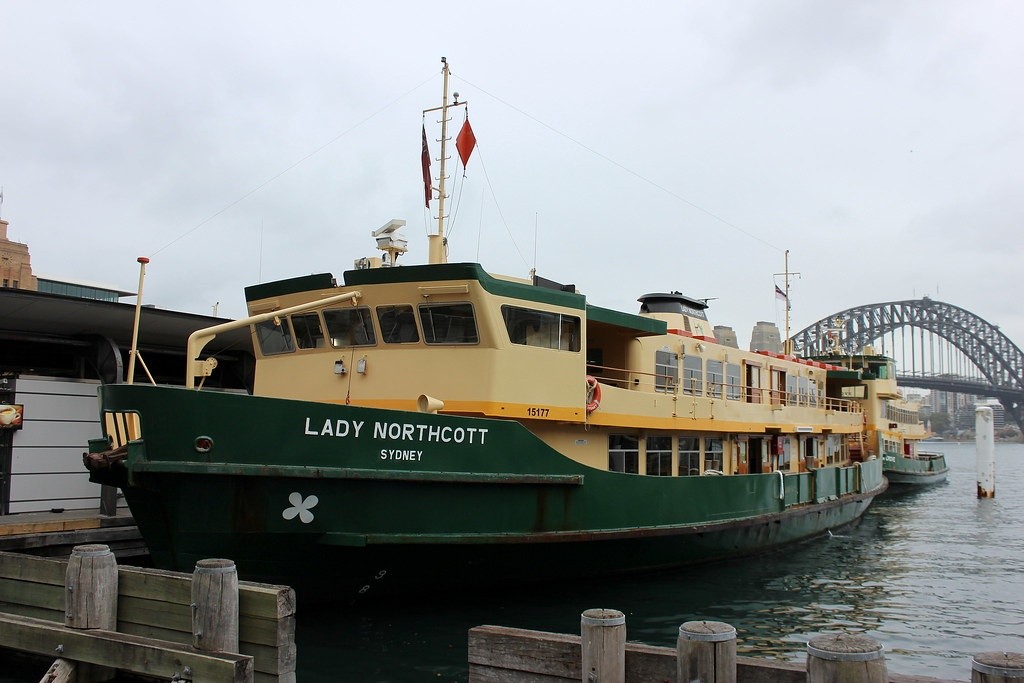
[586,374,602,412]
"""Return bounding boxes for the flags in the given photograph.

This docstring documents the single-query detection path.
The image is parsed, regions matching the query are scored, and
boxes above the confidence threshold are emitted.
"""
[775,285,786,301]
[421,124,432,208]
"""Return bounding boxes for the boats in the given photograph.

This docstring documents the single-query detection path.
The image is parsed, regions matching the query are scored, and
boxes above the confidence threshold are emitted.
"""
[796,319,952,499]
[81,53,890,623]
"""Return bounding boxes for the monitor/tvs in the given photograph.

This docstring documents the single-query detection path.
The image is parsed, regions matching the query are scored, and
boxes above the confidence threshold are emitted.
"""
[0,403,24,430]
